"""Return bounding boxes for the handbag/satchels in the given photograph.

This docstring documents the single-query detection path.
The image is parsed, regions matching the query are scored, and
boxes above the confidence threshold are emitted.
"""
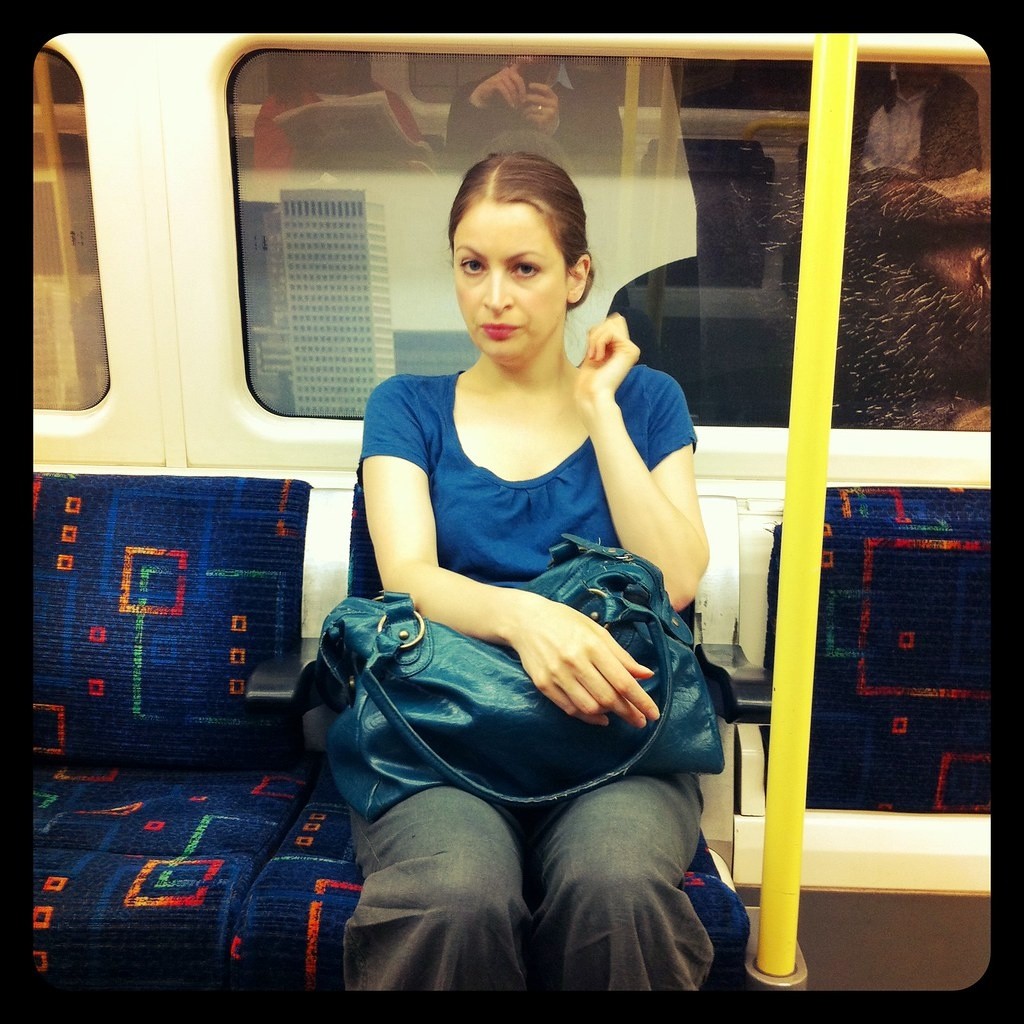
[316,535,723,820]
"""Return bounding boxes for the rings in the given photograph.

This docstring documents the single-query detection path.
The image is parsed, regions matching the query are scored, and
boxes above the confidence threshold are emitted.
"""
[539,105,543,115]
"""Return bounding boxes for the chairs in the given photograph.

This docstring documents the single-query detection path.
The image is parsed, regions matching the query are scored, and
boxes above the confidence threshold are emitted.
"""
[14,473,992,992]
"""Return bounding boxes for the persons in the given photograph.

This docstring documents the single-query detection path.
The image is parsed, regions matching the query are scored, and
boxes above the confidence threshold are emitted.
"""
[798,62,986,182]
[342,153,714,990]
[253,49,623,199]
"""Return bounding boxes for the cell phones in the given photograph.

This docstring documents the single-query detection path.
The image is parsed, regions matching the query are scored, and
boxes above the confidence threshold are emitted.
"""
[496,64,549,113]
